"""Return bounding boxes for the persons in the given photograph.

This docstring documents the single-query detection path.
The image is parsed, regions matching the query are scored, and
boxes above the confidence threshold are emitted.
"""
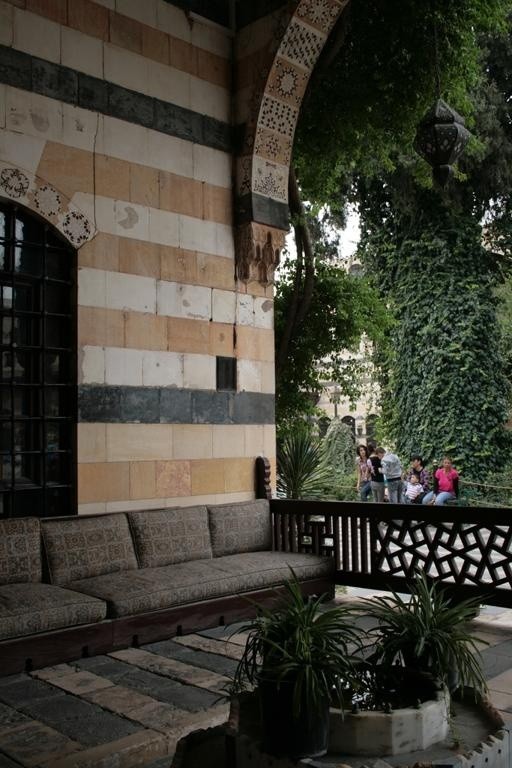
[354,441,460,506]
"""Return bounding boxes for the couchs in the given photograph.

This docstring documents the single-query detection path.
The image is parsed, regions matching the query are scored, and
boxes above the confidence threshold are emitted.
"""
[0,495,334,683]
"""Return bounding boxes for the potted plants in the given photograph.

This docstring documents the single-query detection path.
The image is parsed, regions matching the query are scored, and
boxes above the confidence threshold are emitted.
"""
[209,564,374,763]
[350,565,496,753]
